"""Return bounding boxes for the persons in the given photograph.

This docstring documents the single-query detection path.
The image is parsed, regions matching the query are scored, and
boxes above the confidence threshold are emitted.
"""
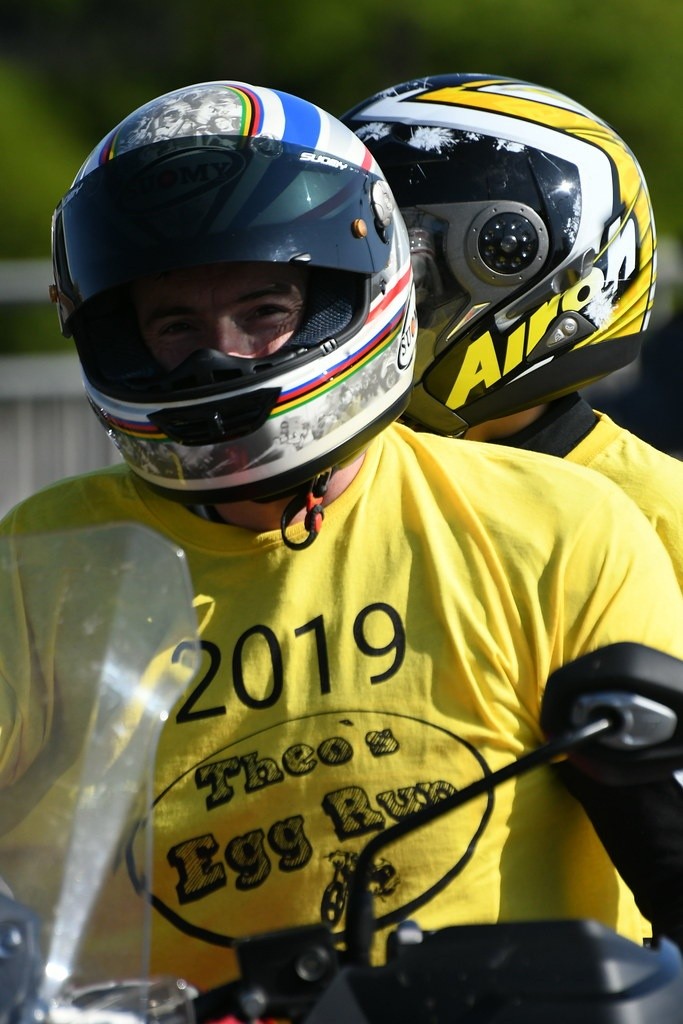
[349,73,683,591]
[0,79,683,991]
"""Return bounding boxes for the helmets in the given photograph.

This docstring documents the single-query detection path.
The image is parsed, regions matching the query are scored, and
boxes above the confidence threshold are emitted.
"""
[49,79,418,550]
[339,71,657,438]
